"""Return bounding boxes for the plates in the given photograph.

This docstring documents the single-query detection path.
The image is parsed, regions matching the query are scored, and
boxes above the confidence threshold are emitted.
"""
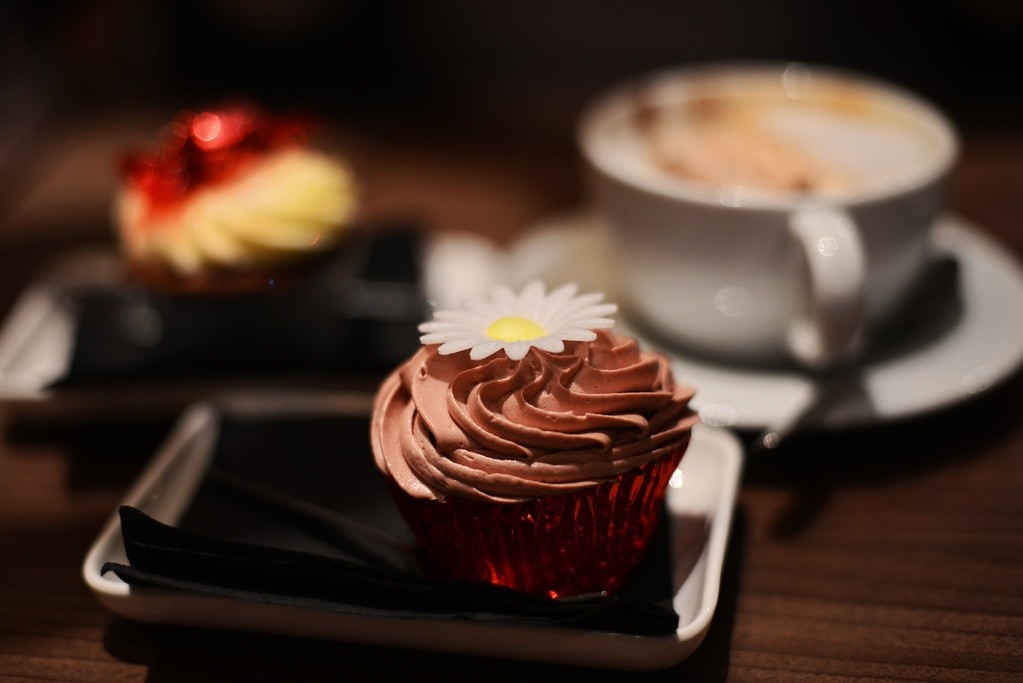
[0,231,425,483]
[73,383,745,678]
[479,199,1023,438]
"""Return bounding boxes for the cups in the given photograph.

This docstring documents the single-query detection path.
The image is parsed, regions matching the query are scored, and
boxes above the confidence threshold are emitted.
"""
[571,60,957,372]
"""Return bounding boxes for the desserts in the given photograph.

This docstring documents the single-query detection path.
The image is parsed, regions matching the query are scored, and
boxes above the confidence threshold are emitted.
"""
[369,284,700,602]
[97,107,361,317]
